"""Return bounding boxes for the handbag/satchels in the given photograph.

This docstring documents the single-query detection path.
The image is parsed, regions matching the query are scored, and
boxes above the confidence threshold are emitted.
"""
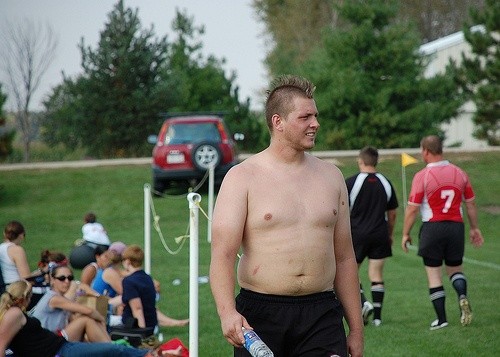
[75,293,108,344]
[110,333,144,347]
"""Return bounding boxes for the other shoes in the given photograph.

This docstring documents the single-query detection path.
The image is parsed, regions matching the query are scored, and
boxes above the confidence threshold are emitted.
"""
[362,302,374,326]
[372,320,383,327]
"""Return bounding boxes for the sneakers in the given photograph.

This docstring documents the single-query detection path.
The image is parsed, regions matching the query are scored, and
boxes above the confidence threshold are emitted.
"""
[430,320,448,330]
[458,296,474,326]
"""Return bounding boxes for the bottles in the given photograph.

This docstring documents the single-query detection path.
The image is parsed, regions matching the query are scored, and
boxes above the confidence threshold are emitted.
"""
[158,331,164,345]
[242,327,274,357]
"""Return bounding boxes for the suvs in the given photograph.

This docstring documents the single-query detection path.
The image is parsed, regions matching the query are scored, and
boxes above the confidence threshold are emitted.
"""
[147,111,244,198]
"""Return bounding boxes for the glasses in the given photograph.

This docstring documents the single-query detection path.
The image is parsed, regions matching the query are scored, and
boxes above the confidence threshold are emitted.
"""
[54,274,75,282]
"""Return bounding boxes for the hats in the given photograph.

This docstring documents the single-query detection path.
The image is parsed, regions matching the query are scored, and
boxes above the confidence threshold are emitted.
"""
[109,241,126,256]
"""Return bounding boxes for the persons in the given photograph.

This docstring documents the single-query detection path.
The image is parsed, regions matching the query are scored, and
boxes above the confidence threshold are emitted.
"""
[402,134,484,328]
[0,211,189,357]
[209,76,364,357]
[338,146,399,326]
